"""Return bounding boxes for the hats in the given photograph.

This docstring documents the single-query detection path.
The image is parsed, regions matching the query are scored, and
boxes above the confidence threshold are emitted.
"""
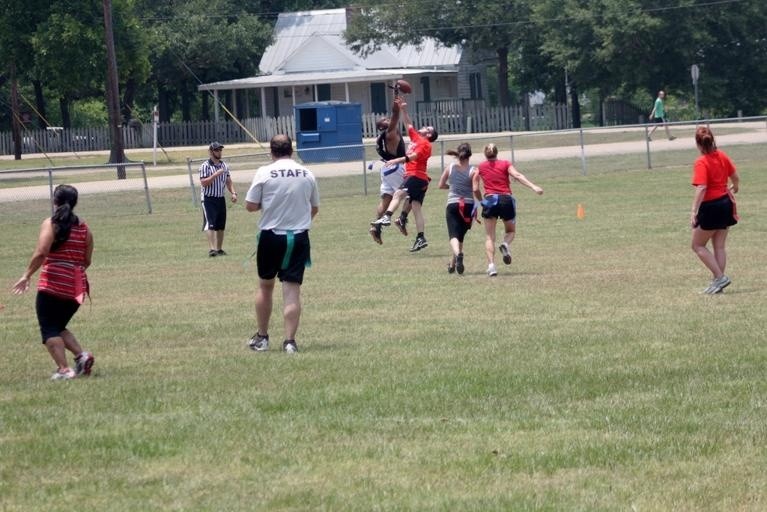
[210,142,224,151]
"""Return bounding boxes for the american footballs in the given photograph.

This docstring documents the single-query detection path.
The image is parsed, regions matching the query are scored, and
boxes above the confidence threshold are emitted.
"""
[395,81,414,97]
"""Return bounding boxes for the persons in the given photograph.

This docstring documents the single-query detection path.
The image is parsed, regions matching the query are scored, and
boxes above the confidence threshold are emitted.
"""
[370,82,416,245]
[197,140,238,257]
[371,95,439,253]
[12,184,94,382]
[690,126,742,294]
[472,141,546,277]
[440,141,478,274]
[244,133,320,356]
[647,90,676,142]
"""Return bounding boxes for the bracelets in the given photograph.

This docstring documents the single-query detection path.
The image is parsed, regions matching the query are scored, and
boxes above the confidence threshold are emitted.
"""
[231,192,237,196]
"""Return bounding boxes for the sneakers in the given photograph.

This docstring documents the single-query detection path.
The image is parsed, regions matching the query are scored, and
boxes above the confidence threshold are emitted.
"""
[283,339,297,352]
[249,333,268,350]
[701,275,731,292]
[51,351,93,379]
[369,215,511,275]
